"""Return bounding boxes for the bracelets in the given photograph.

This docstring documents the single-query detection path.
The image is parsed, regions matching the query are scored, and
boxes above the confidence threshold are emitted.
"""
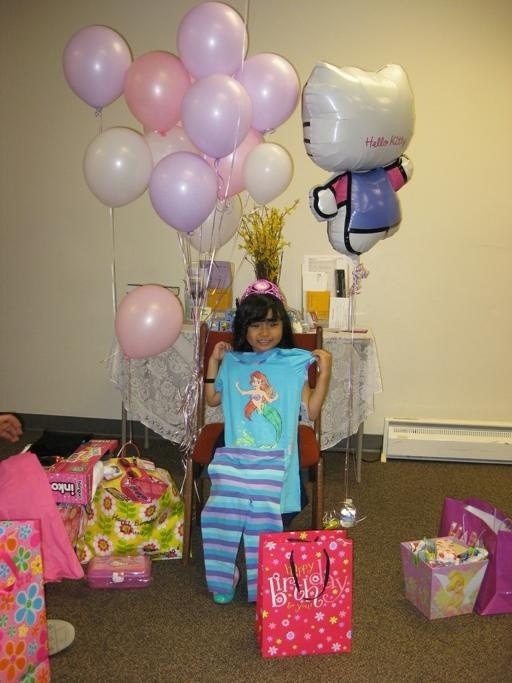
[204,377,216,383]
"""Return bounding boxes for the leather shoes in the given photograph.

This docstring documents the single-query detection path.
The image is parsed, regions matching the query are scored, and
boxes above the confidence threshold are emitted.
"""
[212,565,240,604]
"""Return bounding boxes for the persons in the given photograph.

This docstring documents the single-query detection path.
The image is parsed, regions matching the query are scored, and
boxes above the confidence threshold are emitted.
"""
[0,412,23,444]
[199,278,332,606]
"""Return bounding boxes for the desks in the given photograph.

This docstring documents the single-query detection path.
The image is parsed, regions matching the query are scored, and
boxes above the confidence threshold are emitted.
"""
[113,320,378,489]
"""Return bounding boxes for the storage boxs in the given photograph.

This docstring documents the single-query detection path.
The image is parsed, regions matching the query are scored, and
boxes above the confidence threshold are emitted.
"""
[18,438,119,505]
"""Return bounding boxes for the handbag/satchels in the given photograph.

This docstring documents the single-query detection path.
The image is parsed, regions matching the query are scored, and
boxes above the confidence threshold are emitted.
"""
[0,518,53,682]
[75,440,193,566]
[438,496,511,616]
[255,527,353,659]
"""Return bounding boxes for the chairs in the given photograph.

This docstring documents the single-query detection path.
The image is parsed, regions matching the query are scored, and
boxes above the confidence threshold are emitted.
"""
[184,322,324,568]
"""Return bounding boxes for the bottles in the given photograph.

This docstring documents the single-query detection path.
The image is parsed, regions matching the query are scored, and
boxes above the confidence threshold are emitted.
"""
[339,502,357,528]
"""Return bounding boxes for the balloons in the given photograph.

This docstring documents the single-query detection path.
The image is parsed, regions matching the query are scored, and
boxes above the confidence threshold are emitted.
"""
[114,284,185,361]
[60,0,293,254]
[301,62,417,259]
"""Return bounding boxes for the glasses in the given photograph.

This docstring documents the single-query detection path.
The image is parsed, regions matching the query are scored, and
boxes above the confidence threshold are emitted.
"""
[117,457,138,481]
